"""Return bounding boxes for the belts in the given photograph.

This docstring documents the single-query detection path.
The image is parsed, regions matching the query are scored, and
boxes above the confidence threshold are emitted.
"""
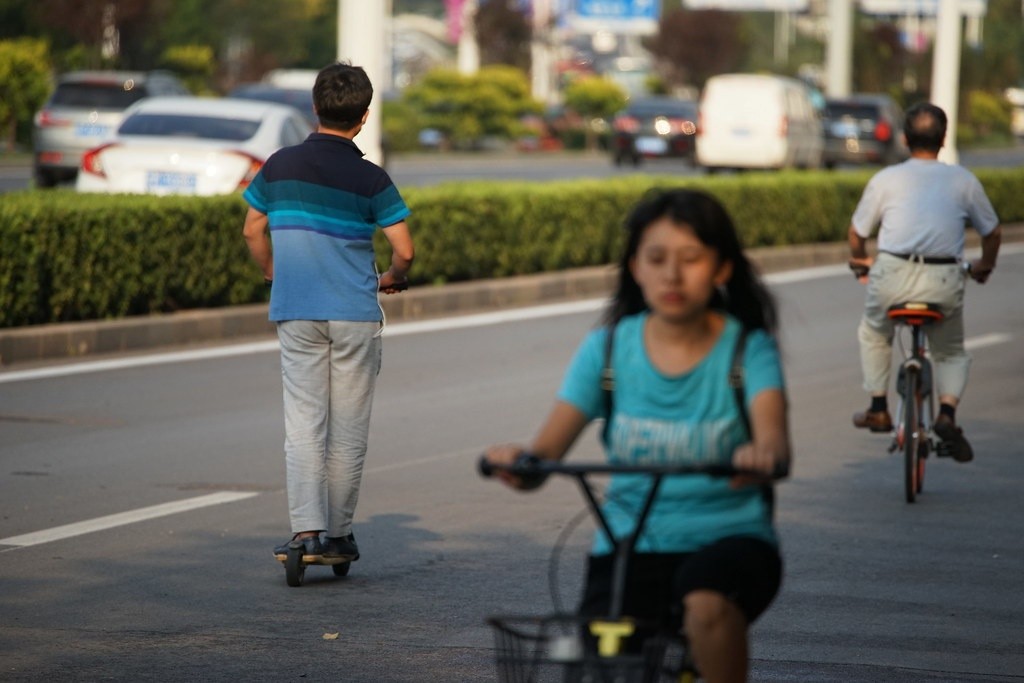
[893,253,957,264]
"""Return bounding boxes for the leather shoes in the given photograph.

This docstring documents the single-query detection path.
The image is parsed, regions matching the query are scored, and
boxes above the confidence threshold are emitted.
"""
[273,535,324,562]
[323,532,360,562]
[853,410,891,431]
[933,415,973,461]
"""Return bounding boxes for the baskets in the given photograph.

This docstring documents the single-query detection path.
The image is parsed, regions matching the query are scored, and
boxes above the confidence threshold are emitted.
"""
[484,609,692,683]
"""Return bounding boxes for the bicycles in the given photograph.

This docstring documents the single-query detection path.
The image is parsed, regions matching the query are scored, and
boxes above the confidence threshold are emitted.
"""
[469,453,789,683]
[853,263,974,502]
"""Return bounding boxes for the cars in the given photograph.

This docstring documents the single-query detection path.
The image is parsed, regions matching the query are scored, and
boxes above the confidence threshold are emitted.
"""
[610,97,702,168]
[69,94,313,198]
[820,91,913,165]
[33,71,194,191]
[231,67,322,125]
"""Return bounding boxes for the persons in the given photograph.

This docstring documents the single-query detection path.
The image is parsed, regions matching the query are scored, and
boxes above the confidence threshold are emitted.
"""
[848,102,1001,462]
[243,64,415,555]
[482,188,786,683]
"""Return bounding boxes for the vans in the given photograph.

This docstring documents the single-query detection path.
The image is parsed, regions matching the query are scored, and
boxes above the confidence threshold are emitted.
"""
[695,72,831,175]
